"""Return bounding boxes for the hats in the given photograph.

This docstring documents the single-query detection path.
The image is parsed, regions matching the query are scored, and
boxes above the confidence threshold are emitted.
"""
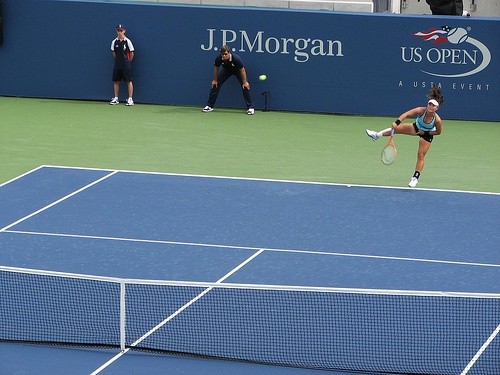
[116,24,126,30]
[428,99,439,108]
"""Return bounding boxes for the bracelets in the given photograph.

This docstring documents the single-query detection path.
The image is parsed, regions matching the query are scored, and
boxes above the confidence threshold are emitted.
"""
[424,131,430,136]
[395,119,401,126]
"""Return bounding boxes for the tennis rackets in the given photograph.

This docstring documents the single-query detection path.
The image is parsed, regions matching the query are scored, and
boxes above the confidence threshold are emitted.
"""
[381,128,397,166]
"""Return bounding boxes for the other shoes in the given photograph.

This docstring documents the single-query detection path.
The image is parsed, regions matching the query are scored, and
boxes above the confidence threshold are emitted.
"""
[110,98,119,104]
[202,106,214,112]
[408,177,418,187]
[247,108,254,115]
[126,97,134,105]
[366,129,379,142]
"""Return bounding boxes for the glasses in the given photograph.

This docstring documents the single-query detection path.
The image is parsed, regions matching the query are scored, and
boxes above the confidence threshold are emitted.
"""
[221,53,228,55]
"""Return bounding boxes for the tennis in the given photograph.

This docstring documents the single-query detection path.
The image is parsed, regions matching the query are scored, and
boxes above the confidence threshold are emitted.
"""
[259,75,266,80]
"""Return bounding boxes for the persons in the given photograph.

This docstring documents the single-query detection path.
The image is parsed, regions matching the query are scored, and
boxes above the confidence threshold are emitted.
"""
[109,24,135,106]
[366,86,442,188]
[202,45,254,115]
